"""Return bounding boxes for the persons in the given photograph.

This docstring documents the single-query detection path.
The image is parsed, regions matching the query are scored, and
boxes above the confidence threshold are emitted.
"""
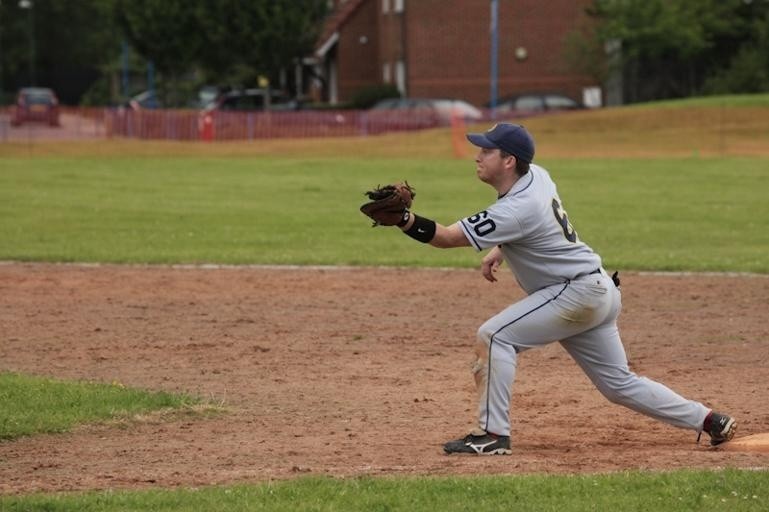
[360,123,737,455]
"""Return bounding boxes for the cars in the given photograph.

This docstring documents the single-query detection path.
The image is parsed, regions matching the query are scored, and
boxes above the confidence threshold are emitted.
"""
[359,91,488,135]
[116,85,234,114]
[482,90,594,120]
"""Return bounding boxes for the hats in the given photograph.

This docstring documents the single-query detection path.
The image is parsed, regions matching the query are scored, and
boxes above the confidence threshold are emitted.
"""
[466,122,535,162]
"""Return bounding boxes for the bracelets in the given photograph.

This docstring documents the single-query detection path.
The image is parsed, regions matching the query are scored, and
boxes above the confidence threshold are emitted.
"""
[403,213,435,243]
[397,210,410,226]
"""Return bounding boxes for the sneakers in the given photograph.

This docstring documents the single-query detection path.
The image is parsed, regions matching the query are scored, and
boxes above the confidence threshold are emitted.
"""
[710,413,737,446]
[444,429,512,455]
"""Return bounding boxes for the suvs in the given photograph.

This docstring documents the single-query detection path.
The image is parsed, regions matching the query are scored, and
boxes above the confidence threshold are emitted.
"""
[9,85,61,128]
[197,89,360,138]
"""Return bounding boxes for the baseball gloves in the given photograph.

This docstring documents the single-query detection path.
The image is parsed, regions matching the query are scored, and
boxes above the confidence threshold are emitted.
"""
[360,183,413,226]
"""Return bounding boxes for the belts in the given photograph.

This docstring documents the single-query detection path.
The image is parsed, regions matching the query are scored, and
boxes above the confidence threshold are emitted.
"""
[590,268,601,274]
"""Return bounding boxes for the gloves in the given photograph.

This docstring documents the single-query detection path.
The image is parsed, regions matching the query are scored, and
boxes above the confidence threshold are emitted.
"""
[612,271,620,286]
